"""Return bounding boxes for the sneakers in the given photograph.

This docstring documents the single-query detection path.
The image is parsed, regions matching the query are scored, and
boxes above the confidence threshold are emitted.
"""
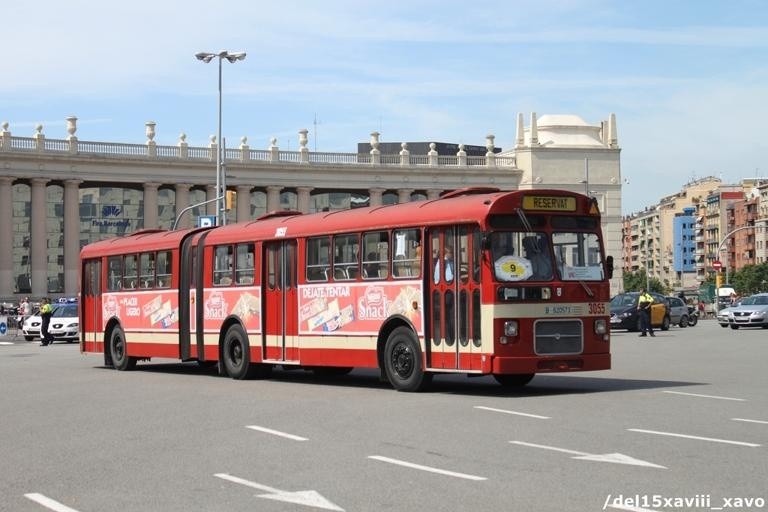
[649,333,656,337]
[40,343,48,346]
[50,336,55,344]
[639,333,648,337]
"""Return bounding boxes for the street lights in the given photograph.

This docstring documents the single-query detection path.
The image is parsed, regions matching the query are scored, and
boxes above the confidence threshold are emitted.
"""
[194,48,249,230]
[578,179,597,267]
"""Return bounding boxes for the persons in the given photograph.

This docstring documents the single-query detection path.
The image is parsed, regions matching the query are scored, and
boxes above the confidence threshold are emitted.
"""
[698,300,705,318]
[17,296,54,346]
[434,245,454,284]
[533,238,557,275]
[637,287,655,337]
[730,293,737,304]
[365,252,380,278]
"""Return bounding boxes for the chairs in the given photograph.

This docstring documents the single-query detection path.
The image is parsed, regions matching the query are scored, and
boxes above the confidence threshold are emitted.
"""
[114,277,172,288]
[345,264,370,279]
[218,275,234,287]
[378,259,391,278]
[394,254,409,278]
[237,275,254,284]
[322,266,347,280]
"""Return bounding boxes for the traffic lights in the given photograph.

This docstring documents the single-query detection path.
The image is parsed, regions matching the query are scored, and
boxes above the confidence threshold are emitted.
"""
[225,190,236,210]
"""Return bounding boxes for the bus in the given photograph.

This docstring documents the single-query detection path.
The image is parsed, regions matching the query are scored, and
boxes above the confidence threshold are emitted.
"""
[78,186,627,397]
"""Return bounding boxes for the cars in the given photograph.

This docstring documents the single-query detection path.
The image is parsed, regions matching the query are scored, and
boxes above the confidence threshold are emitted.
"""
[609,290,671,334]
[663,296,691,329]
[716,297,750,328]
[0,299,80,345]
[726,293,768,329]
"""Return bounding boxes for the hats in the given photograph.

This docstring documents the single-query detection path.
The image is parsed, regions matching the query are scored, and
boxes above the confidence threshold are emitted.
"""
[39,297,47,302]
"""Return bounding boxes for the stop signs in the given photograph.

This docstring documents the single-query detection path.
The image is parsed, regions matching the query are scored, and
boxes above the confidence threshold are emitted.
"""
[710,261,722,271]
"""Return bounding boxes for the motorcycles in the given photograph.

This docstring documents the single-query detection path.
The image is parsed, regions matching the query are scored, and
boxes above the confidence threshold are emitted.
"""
[686,305,700,327]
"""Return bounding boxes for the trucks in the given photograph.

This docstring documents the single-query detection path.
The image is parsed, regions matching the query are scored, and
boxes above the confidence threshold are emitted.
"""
[699,283,739,314]
